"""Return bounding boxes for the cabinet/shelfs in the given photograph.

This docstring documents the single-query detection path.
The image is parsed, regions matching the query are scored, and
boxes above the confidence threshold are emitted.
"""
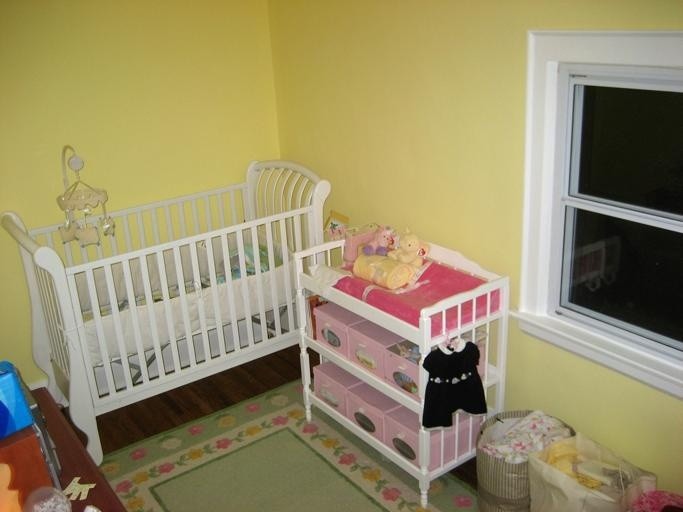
[293,234,509,507]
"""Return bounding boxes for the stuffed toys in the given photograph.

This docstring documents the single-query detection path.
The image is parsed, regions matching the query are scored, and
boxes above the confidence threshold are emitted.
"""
[388,234,429,268]
[365,225,394,257]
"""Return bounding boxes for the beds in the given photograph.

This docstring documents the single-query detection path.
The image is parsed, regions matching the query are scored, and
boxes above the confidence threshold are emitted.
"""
[3,159,331,468]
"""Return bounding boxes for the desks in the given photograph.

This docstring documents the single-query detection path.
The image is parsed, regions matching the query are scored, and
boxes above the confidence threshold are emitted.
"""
[31,387,124,512]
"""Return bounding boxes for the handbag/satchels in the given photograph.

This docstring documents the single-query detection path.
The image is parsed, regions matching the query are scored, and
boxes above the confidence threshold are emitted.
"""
[475,408,577,512]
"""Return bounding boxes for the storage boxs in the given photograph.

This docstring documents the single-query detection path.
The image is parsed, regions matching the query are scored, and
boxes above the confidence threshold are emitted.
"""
[349,322,403,379]
[311,362,361,414]
[344,383,401,441]
[384,341,484,403]
[313,302,365,358]
[385,410,483,470]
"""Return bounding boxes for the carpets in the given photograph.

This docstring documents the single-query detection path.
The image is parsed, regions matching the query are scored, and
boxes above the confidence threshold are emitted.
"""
[101,379,478,512]
